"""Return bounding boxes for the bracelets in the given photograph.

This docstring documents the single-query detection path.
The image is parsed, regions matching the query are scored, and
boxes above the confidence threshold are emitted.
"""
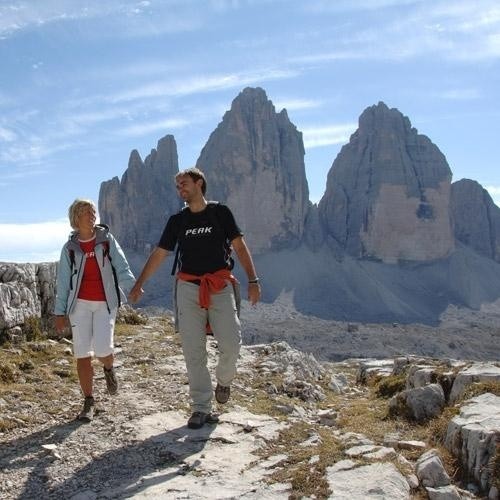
[247,278,260,284]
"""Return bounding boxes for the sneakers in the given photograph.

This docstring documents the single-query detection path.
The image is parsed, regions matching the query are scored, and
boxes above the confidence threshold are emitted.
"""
[102,366,119,395]
[215,382,231,404]
[78,396,101,421]
[187,412,212,430]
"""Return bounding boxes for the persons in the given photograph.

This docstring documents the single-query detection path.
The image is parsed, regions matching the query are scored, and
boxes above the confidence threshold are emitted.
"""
[126,168,262,430]
[52,199,145,422]
